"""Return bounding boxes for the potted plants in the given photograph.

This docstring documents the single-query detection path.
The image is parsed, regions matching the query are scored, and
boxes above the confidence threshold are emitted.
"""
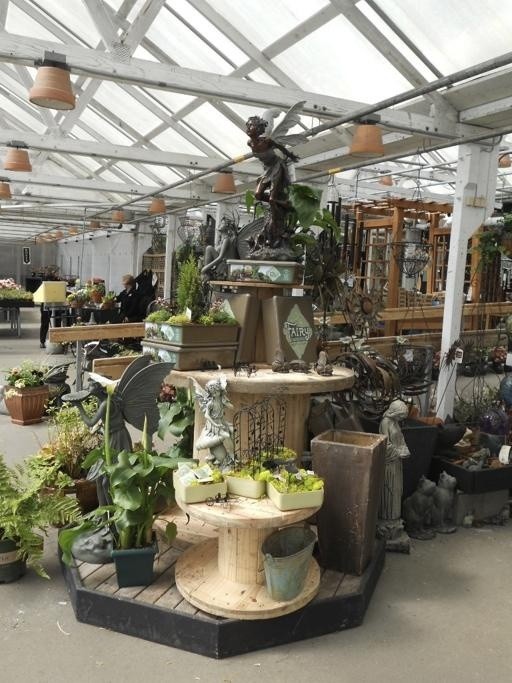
[39,395,104,528]
[2,360,48,426]
[67,277,116,310]
[1,454,83,583]
[80,383,174,590]
[42,361,76,407]
[144,254,240,345]
[0,276,34,306]
[474,202,512,263]
[176,444,325,512]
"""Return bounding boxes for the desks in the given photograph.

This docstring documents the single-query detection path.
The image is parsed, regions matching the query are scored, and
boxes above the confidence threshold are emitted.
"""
[170,490,327,624]
[170,362,356,469]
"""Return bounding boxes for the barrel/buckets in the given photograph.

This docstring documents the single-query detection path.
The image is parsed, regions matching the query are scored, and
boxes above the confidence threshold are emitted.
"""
[258,526,316,601]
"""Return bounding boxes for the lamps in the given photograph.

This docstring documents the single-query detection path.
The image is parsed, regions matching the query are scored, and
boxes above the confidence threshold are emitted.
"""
[209,167,237,195]
[0,181,11,199]
[2,146,34,172]
[34,194,169,246]
[351,121,385,158]
[28,58,75,112]
[389,167,434,280]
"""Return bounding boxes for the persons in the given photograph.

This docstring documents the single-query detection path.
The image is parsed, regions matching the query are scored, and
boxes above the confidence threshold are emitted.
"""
[244,114,301,246]
[112,271,144,323]
[376,396,413,521]
[37,262,62,348]
[71,379,135,540]
[204,214,240,283]
[195,375,235,461]
